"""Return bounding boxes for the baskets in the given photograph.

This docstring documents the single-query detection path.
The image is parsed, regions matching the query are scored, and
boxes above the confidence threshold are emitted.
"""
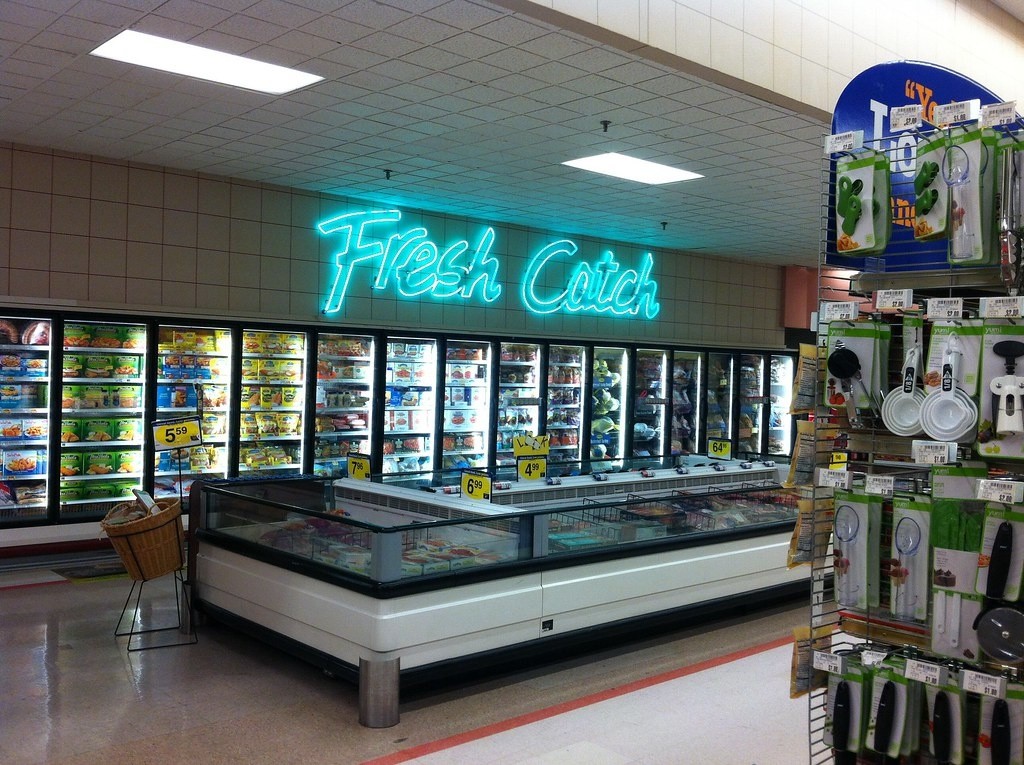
[99,498,186,581]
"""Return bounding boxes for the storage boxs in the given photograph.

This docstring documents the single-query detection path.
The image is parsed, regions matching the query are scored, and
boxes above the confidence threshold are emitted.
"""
[310,509,687,577]
[0,322,486,502]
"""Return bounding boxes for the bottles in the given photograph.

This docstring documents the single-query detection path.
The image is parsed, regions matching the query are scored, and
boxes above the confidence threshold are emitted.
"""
[548,366,580,383]
